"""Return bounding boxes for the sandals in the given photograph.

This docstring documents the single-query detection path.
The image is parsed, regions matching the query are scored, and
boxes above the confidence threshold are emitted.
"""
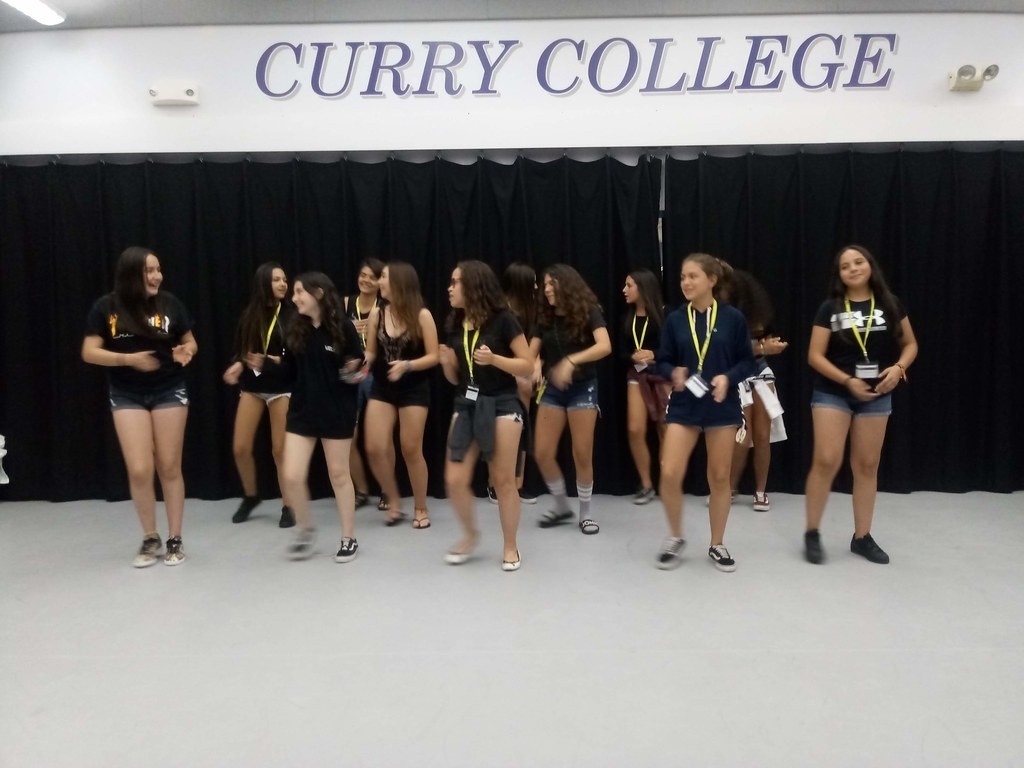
[387,510,406,526]
[412,507,430,529]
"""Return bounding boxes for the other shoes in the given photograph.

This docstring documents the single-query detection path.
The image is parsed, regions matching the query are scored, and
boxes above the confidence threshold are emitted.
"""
[378,494,391,510]
[502,549,521,571]
[355,491,370,509]
[444,530,481,563]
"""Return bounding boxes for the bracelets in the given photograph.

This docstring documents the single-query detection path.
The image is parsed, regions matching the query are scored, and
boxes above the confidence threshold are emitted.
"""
[760,344,764,355]
[845,377,854,384]
[896,363,906,381]
[566,356,575,365]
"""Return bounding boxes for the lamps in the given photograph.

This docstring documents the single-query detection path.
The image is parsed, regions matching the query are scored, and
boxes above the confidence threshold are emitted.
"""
[949,64,1000,90]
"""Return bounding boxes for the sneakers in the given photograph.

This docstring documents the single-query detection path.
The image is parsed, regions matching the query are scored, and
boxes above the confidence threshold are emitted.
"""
[232,496,261,523]
[706,544,736,572]
[279,504,296,528]
[753,492,770,510]
[851,532,889,564]
[164,535,186,566]
[487,485,498,505]
[804,528,822,563]
[517,487,537,504]
[132,531,163,567]
[287,527,318,557]
[335,536,359,562]
[632,488,655,504]
[655,537,687,569]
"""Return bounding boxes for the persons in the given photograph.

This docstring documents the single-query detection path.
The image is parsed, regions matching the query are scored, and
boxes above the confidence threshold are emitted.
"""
[365,261,438,528]
[438,260,534,571]
[82,246,198,566]
[706,269,789,510]
[487,263,541,503]
[656,254,756,573]
[622,269,672,504]
[244,271,366,563]
[804,247,918,563]
[530,264,611,535]
[223,263,296,528]
[341,258,392,510]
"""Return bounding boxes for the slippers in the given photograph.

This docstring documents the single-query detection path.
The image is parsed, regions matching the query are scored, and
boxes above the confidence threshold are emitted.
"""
[537,510,572,528]
[579,519,599,534]
